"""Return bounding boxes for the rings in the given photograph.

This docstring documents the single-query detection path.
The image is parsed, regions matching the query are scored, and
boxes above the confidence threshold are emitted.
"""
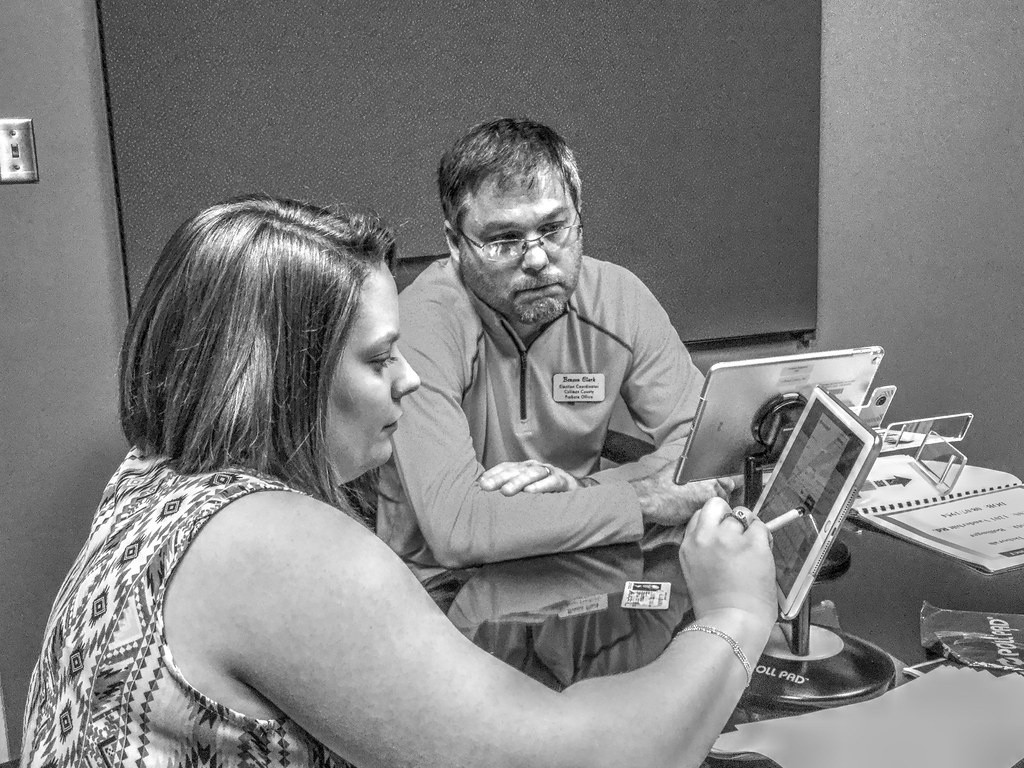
[542,465,551,477]
[725,510,748,531]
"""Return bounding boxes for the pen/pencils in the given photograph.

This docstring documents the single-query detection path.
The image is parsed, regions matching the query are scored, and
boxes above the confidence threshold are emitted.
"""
[765,505,807,533]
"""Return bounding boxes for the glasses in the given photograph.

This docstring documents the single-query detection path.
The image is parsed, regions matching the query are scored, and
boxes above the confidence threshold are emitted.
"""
[458,209,583,262]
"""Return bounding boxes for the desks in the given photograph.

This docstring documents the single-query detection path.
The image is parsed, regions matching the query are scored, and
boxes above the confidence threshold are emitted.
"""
[417,515,1024,768]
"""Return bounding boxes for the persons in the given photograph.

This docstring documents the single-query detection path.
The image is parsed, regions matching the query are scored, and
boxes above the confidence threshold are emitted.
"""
[374,115,746,568]
[18,193,776,768]
[423,527,686,692]
[634,583,659,590]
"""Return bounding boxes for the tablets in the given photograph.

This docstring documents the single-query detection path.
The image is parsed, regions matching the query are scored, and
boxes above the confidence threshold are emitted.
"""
[750,383,881,620]
[676,347,882,484]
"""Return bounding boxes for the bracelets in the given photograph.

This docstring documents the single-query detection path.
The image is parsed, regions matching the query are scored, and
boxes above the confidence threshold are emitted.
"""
[676,625,752,689]
